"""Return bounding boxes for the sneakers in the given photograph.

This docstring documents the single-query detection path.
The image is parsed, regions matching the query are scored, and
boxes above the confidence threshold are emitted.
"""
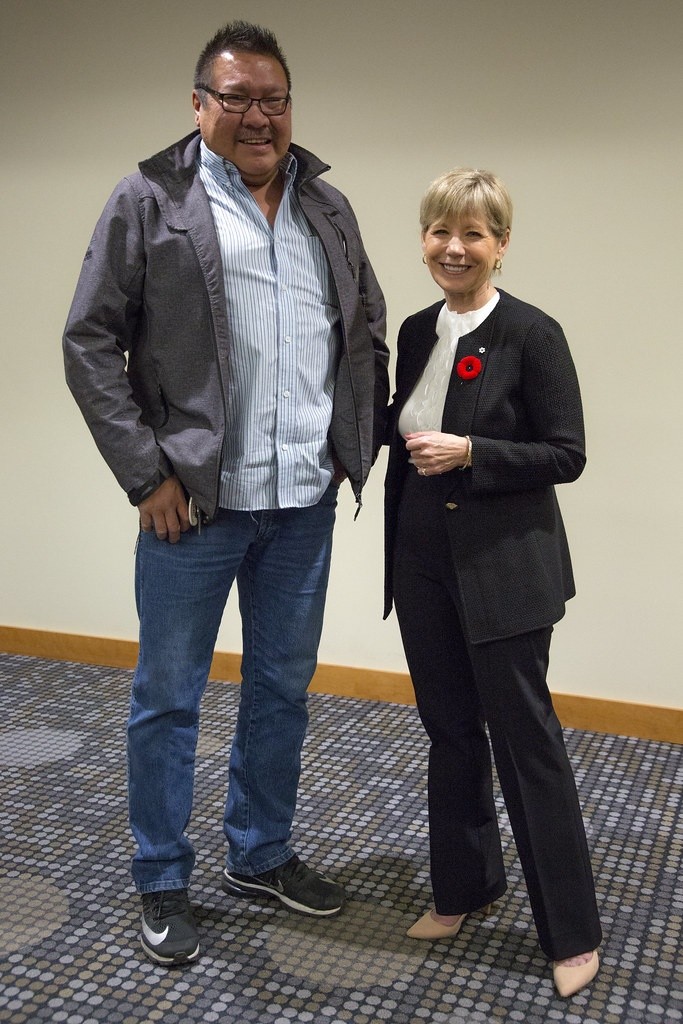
[220,853,345,918]
[139,887,201,966]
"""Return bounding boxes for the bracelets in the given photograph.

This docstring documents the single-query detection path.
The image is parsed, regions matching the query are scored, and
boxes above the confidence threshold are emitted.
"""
[459,435,472,470]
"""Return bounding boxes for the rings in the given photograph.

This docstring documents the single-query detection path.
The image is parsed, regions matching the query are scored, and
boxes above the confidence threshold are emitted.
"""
[422,468,428,476]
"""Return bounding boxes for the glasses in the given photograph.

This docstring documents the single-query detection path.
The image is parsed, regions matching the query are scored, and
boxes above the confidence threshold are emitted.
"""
[195,83,291,116]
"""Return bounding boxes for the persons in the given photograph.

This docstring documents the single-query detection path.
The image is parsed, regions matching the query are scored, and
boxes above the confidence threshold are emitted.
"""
[378,165,601,997]
[61,21,388,969]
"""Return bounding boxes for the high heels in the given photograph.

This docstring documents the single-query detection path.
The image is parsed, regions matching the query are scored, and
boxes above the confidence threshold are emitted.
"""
[406,900,492,940]
[552,946,599,998]
